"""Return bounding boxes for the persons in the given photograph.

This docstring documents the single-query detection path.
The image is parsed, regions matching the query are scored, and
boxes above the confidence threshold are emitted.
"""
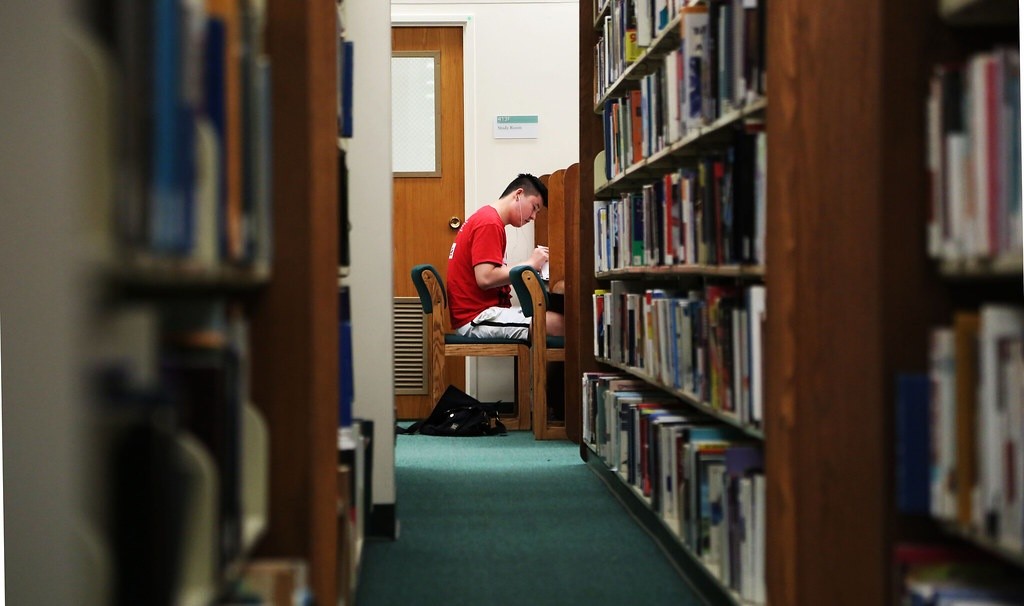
[447,172,565,340]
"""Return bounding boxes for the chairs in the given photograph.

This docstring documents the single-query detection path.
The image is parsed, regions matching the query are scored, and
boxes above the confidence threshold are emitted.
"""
[410,264,532,432]
[508,264,568,440]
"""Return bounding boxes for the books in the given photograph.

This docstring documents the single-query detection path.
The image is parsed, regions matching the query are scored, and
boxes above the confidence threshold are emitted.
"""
[880,0,1020,606]
[580,0,772,606]
[71,0,314,606]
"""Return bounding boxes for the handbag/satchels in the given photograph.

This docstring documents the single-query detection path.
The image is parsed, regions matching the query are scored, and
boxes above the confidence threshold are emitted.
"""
[397,385,508,436]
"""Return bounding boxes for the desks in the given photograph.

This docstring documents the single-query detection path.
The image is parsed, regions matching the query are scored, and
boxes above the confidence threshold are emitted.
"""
[532,163,582,444]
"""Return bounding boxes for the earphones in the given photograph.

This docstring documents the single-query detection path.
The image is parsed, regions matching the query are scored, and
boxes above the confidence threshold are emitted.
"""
[517,194,519,199]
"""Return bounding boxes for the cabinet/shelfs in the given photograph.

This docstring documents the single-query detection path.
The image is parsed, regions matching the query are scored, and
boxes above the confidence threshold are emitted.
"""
[575,0,898,606]
